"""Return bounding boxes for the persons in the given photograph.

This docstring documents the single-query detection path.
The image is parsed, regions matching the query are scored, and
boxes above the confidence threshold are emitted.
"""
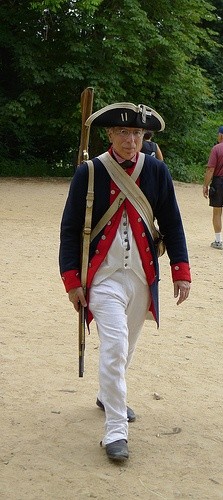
[202,126,223,249]
[59,102,193,464]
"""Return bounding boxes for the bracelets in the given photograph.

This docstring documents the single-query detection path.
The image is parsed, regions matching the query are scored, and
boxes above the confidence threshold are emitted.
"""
[203,187,208,188]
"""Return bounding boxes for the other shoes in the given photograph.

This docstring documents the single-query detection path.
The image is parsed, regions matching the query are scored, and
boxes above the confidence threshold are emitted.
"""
[210,240,223,249]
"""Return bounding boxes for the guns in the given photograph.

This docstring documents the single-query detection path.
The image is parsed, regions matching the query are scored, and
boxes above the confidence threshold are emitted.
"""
[76,83,96,378]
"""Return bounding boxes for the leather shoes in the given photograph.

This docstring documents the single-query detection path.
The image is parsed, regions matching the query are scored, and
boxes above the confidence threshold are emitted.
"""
[105,439,129,460]
[96,397,136,422]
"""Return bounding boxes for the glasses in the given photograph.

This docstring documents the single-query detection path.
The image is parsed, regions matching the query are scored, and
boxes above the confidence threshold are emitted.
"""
[113,129,144,136]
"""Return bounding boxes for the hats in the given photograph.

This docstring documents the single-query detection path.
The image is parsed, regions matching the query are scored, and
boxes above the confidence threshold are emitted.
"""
[84,102,165,132]
[215,126,223,134]
